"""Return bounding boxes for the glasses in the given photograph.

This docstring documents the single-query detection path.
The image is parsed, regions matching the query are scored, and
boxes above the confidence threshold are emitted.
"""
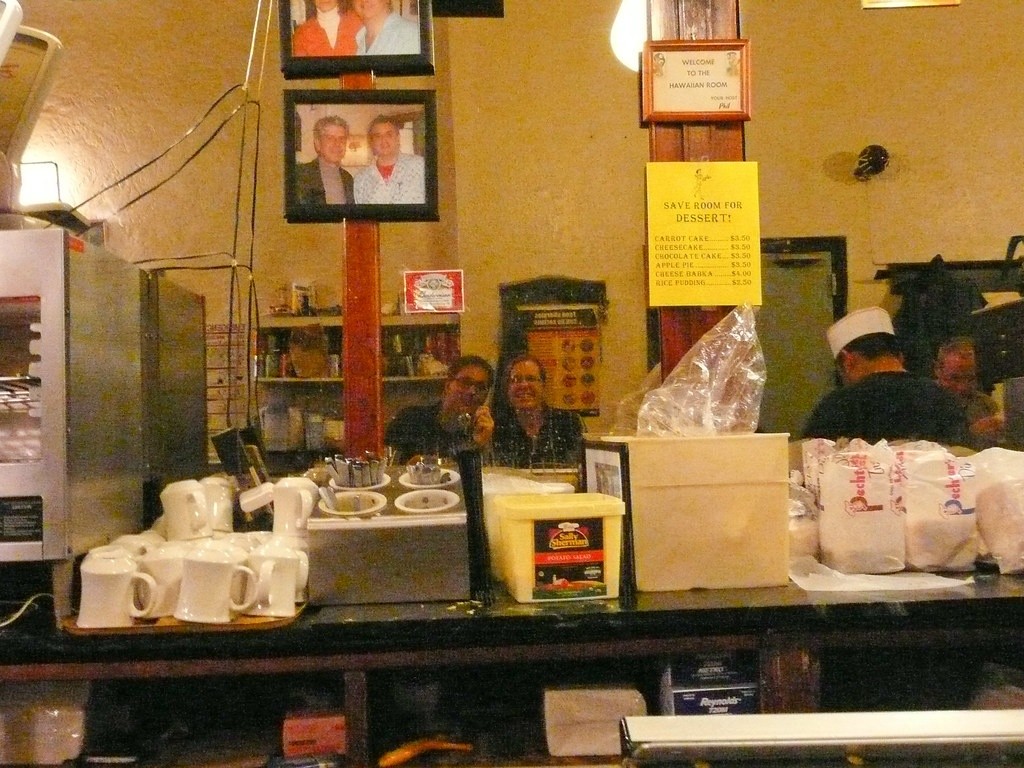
[507,374,544,384]
[450,374,493,392]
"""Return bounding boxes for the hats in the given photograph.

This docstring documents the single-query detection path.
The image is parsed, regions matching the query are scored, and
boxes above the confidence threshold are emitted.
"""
[826,306,895,359]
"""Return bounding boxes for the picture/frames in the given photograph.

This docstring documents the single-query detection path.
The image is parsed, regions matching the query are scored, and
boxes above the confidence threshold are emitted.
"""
[641,38,752,123]
[277,0,435,74]
[281,87,441,223]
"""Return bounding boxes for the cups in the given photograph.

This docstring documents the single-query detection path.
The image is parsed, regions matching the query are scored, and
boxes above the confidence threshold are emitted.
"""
[319,469,461,516]
[76,477,318,624]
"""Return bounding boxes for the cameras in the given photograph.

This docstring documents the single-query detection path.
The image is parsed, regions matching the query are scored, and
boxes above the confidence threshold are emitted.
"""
[458,412,477,429]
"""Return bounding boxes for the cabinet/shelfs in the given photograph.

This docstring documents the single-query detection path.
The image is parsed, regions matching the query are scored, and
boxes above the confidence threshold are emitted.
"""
[256,311,460,387]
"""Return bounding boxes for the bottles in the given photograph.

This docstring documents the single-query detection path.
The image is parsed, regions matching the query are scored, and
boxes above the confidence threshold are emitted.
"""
[386,328,461,377]
[260,380,345,451]
[265,328,342,379]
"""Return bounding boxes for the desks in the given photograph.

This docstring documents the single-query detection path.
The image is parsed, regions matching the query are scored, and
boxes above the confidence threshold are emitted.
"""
[0,564,1024,768]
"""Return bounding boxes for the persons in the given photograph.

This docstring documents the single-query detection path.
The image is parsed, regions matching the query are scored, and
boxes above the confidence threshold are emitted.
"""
[800,302,970,446]
[352,111,426,206]
[290,115,356,206]
[922,331,1006,440]
[381,351,494,505]
[291,1,365,57]
[486,353,587,471]
[350,0,422,55]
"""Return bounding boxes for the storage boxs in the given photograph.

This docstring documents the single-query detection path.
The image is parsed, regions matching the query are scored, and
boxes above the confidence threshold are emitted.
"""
[282,704,347,760]
[494,491,628,603]
[657,686,762,716]
[657,651,760,691]
[583,431,791,592]
